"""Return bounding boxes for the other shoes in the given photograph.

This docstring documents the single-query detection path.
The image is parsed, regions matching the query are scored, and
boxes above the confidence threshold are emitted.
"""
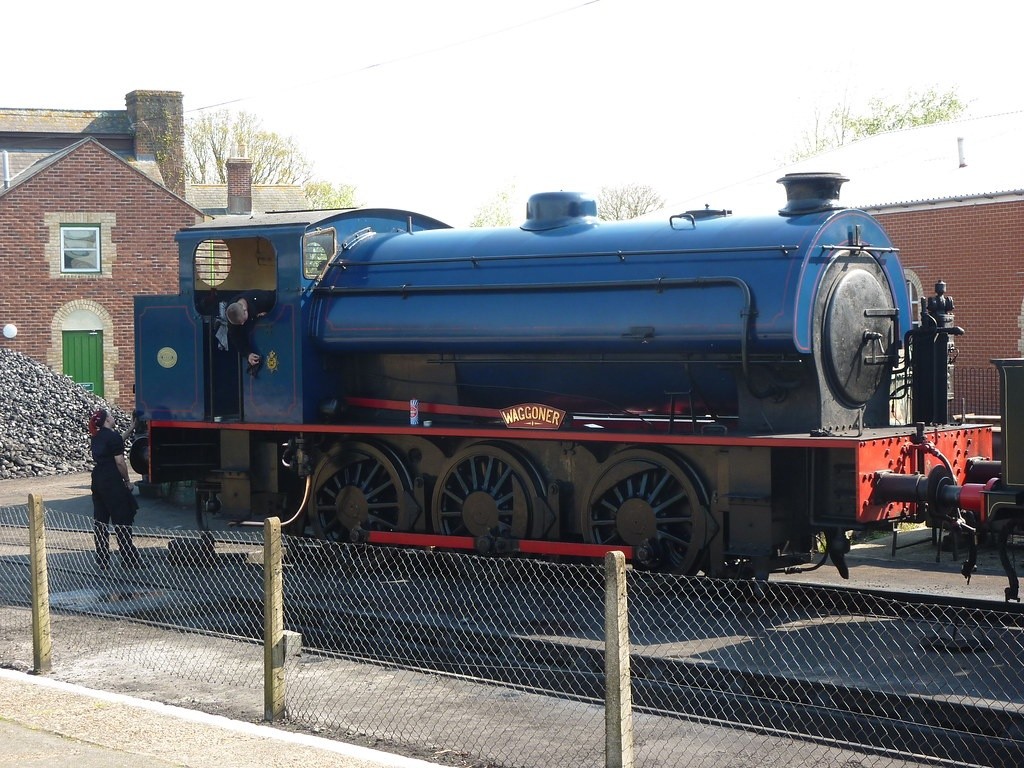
[96,562,110,569]
[120,559,151,569]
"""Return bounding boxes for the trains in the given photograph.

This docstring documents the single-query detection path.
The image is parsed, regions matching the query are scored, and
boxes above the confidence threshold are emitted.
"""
[128,170,1024,612]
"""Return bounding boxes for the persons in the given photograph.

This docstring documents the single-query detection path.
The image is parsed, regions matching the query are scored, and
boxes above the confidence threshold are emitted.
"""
[227,289,274,366]
[88,408,148,567]
